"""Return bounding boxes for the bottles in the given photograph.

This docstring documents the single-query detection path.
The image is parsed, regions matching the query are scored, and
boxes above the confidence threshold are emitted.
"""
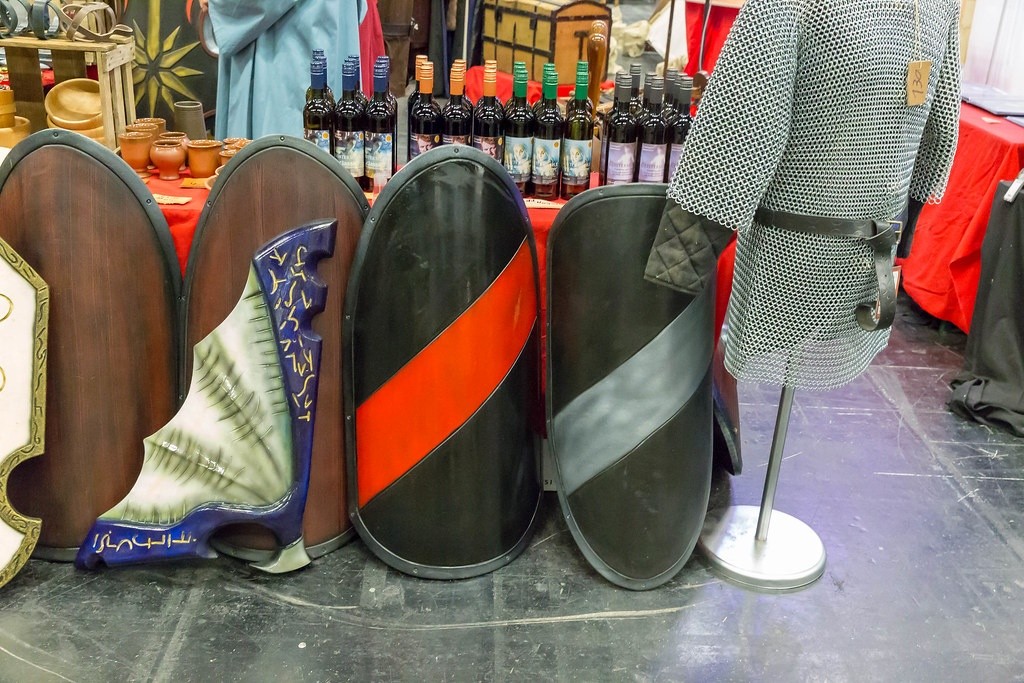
[303,48,693,200]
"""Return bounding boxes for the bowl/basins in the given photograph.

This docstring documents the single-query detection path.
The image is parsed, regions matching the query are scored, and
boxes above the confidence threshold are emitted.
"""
[44,78,104,145]
[0,115,31,148]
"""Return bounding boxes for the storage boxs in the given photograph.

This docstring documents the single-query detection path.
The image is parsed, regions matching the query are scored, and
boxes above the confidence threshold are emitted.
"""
[482,0,613,85]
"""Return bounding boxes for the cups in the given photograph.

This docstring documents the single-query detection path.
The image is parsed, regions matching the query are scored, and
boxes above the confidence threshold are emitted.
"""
[118,101,253,179]
[0,89,16,128]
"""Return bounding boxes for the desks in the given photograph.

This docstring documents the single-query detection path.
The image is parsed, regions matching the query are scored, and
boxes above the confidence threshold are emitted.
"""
[138,173,571,503]
[464,66,617,107]
[891,100,1024,334]
